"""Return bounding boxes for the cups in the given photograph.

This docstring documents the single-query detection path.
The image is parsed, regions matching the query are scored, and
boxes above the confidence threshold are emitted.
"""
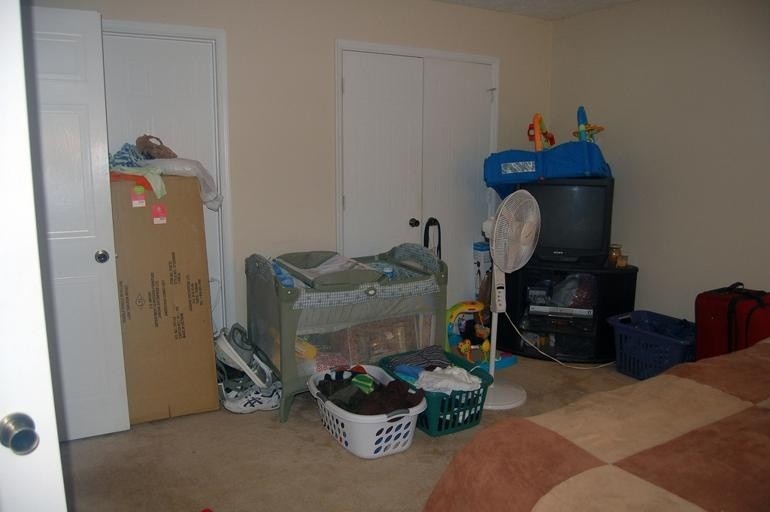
[549,334,556,347]
[616,255,628,270]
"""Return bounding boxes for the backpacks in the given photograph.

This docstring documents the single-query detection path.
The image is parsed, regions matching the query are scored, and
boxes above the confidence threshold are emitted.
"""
[208,325,285,401]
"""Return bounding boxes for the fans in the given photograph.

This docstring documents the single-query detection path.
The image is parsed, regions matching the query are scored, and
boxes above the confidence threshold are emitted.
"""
[476,188,545,410]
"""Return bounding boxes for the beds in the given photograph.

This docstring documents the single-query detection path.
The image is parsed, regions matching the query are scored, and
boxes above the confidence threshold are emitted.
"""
[245,242,449,423]
[420,336,769,512]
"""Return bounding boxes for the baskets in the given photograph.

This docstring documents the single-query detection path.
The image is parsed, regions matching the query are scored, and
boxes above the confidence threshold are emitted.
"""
[605,310,696,383]
[376,343,495,437]
[305,360,428,460]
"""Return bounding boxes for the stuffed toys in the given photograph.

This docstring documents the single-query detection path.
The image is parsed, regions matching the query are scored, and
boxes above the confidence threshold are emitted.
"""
[446,302,517,373]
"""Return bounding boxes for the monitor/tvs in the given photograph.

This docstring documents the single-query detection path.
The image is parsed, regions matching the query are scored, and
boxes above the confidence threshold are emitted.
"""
[516,177,615,264]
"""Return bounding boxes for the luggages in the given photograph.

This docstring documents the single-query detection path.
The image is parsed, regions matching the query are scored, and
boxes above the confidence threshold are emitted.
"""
[692,281,769,361]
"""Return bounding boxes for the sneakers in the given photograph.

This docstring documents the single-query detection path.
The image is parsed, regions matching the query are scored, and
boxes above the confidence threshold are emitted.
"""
[221,386,283,415]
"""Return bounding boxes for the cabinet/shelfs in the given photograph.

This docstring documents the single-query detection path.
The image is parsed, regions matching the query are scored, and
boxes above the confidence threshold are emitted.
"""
[489,259,641,367]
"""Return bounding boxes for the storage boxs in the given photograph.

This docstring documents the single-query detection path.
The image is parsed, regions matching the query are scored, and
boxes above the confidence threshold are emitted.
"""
[107,173,223,430]
